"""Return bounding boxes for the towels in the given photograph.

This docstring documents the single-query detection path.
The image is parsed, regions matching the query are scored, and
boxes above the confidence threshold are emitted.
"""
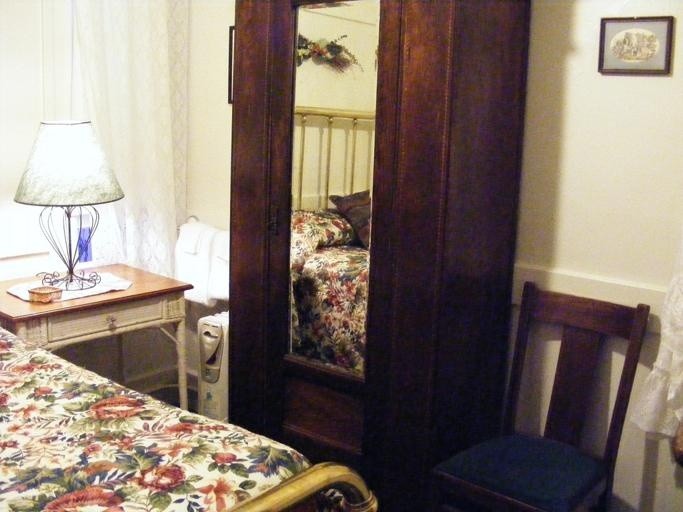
[173,219,231,312]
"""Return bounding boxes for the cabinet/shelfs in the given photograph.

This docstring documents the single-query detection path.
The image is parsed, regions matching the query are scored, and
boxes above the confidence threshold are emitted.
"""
[226,1,534,501]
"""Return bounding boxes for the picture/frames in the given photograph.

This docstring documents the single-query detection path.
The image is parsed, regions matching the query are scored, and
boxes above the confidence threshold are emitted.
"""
[597,14,676,76]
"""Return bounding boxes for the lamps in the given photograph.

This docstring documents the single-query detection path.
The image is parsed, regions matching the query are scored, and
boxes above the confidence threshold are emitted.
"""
[11,117,127,297]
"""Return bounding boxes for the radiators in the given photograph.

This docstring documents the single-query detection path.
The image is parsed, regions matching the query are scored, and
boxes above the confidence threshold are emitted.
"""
[195,310,230,426]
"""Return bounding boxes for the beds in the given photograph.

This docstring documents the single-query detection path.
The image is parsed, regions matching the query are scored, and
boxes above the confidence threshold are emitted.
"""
[0,323,383,512]
[288,106,376,380]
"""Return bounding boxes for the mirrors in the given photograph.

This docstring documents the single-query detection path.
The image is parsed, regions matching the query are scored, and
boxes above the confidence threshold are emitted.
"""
[287,1,384,382]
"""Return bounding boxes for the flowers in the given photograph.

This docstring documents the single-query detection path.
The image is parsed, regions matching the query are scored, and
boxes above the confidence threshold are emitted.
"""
[295,25,368,75]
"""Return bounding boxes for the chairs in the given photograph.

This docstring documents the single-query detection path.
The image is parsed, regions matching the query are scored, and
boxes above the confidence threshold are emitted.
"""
[426,278,652,511]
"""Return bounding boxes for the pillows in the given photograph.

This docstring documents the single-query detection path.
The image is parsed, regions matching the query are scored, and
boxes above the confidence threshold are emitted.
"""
[326,185,372,251]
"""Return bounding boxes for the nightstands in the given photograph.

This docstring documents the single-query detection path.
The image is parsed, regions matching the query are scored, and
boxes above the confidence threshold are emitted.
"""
[0,260,194,414]
